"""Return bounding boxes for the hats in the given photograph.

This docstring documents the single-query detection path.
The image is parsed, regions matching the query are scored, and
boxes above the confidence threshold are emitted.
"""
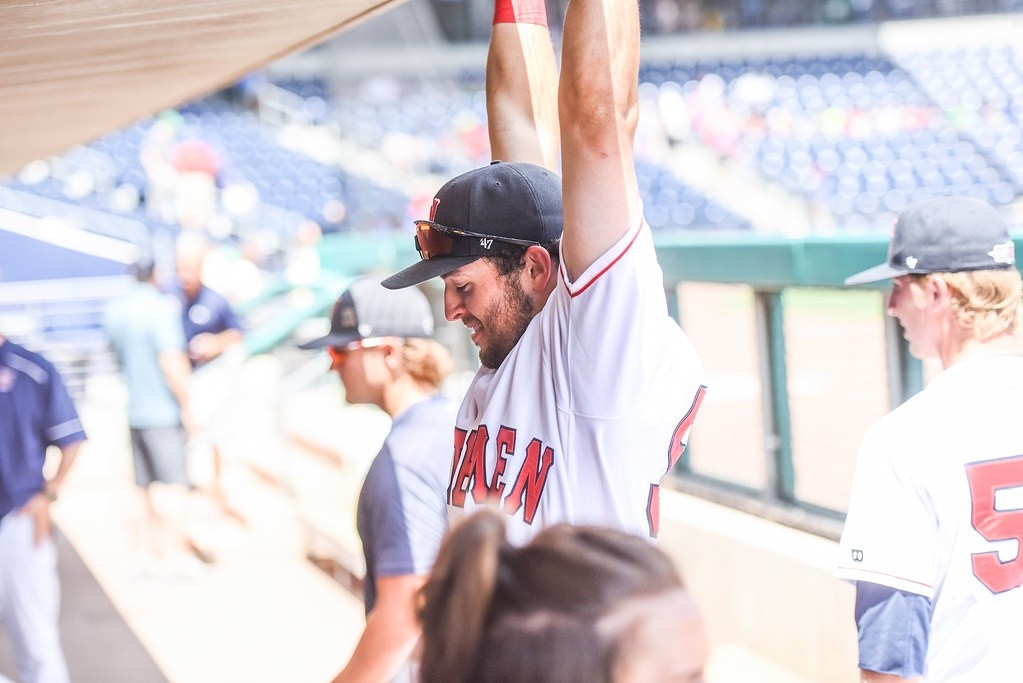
[296,274,434,349]
[380,159,562,289]
[843,193,1014,287]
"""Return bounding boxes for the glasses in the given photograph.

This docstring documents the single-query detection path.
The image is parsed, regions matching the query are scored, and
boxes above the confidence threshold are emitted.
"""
[413,219,541,261]
[327,337,403,361]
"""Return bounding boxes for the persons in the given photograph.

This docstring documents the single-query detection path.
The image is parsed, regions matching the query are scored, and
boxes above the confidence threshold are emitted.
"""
[383,0,710,544]
[842,196,1022,682]
[410,508,709,683]
[0,333,88,683]
[106,228,241,563]
[296,273,457,682]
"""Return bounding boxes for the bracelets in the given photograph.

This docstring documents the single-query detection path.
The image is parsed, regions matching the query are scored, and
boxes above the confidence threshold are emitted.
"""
[493,0,548,27]
[42,489,56,501]
